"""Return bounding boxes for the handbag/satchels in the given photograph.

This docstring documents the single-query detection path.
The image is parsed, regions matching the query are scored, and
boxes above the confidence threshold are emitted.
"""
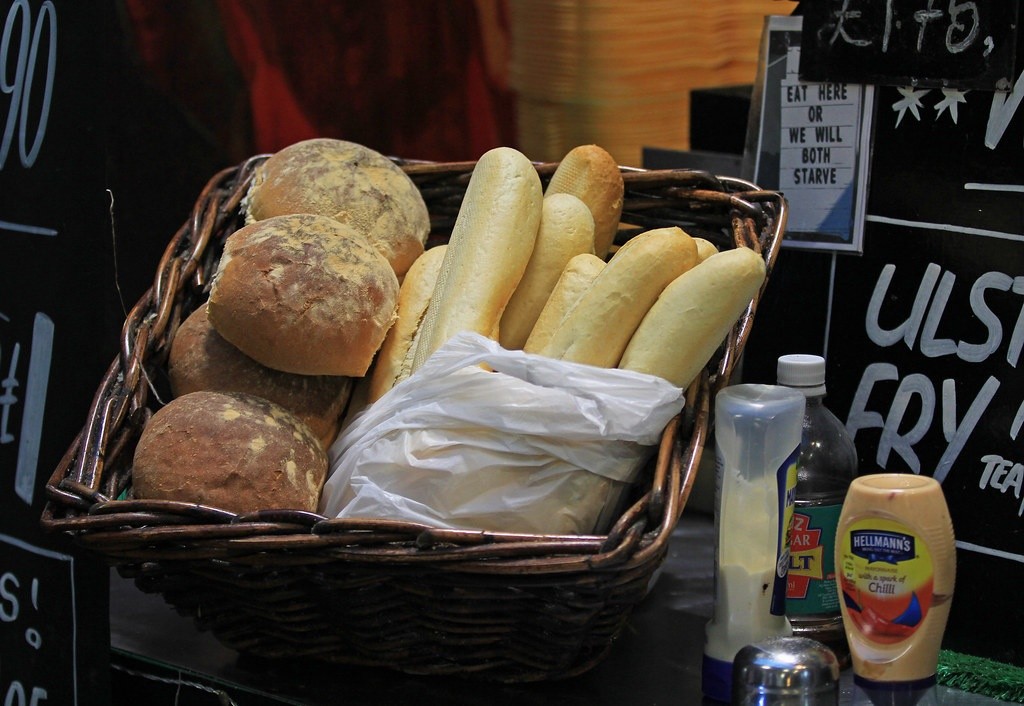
[317,332,686,539]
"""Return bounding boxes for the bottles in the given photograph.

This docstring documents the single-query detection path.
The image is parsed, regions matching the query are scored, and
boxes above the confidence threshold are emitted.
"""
[776,354,858,671]
[701,383,805,701]
[834,473,957,706]
[732,637,840,706]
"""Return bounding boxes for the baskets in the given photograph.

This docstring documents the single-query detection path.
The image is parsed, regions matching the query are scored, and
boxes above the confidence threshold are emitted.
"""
[36,155,788,706]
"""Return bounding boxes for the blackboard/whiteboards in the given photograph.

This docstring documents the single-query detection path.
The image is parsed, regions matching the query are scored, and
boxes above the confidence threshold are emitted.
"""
[820,85,1024,563]
[800,1,1024,91]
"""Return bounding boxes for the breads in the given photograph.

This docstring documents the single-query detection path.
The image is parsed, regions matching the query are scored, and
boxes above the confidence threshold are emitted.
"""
[130,138,429,514]
[339,145,766,535]
